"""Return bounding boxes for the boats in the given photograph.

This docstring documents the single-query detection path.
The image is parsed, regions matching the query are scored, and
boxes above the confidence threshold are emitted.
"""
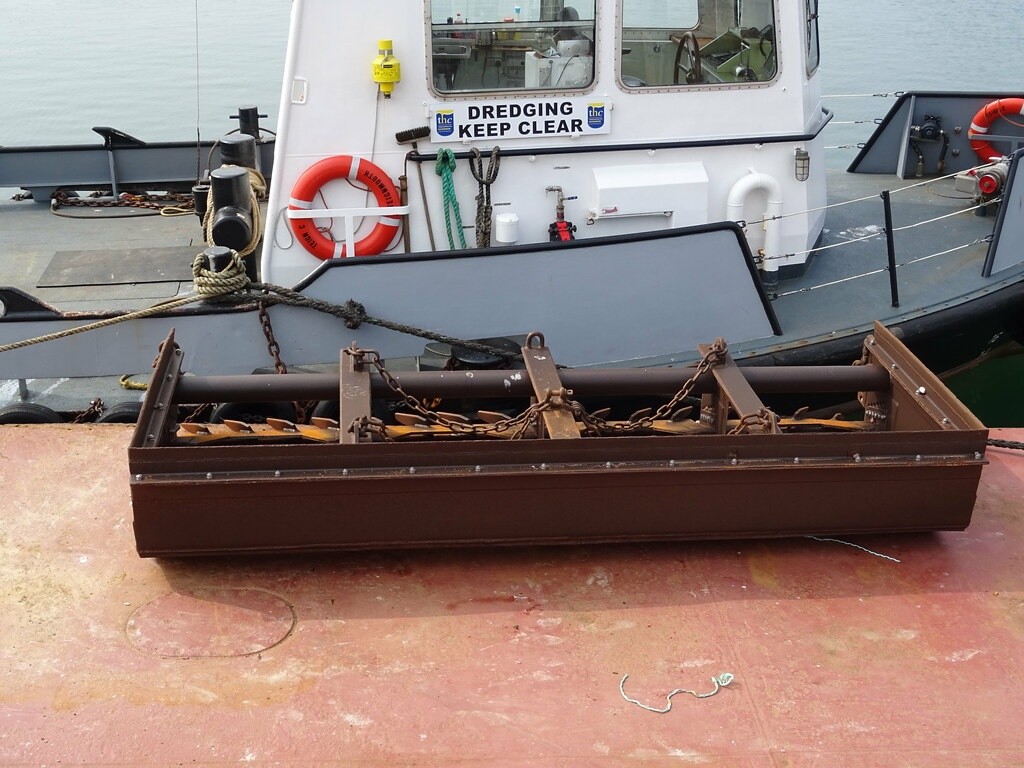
[0,0,1023,421]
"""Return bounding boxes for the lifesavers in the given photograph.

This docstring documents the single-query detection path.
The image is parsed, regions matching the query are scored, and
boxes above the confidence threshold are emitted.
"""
[967,97,1024,163]
[288,155,401,261]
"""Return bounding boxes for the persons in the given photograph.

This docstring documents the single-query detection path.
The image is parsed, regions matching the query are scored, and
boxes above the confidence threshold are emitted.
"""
[554,6,594,56]
